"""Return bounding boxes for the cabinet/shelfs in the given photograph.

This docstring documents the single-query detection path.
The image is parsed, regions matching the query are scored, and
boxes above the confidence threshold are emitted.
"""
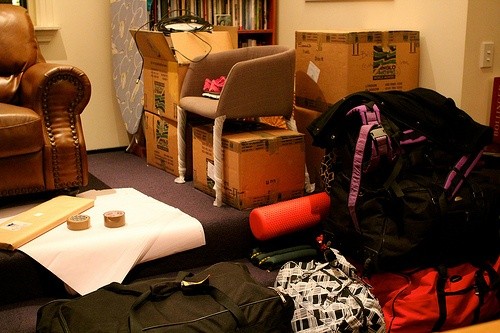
[151,0,279,46]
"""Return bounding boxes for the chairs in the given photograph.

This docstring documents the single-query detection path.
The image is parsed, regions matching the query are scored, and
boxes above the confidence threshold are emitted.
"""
[173,44,295,208]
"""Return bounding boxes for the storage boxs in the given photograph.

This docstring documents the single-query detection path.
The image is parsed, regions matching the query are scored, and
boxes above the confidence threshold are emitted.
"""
[128,27,420,211]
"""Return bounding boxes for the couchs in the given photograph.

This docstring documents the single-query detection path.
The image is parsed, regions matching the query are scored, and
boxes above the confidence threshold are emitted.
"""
[0,5,92,199]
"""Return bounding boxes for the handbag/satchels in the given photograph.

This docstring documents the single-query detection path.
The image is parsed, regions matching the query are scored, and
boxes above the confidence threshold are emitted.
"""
[36,102,500,333]
